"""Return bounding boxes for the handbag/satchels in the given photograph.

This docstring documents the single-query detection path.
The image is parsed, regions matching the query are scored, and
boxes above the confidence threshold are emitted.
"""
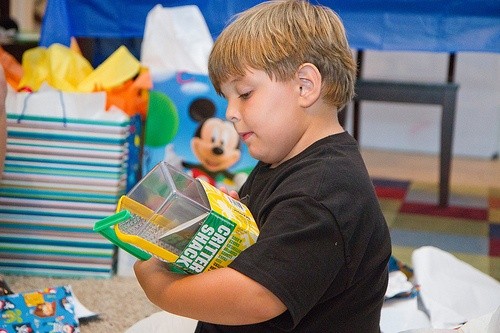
[141,70,259,198]
[0,87,130,280]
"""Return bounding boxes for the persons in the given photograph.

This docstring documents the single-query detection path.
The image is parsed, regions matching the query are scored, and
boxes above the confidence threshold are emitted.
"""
[131,1,392,333]
